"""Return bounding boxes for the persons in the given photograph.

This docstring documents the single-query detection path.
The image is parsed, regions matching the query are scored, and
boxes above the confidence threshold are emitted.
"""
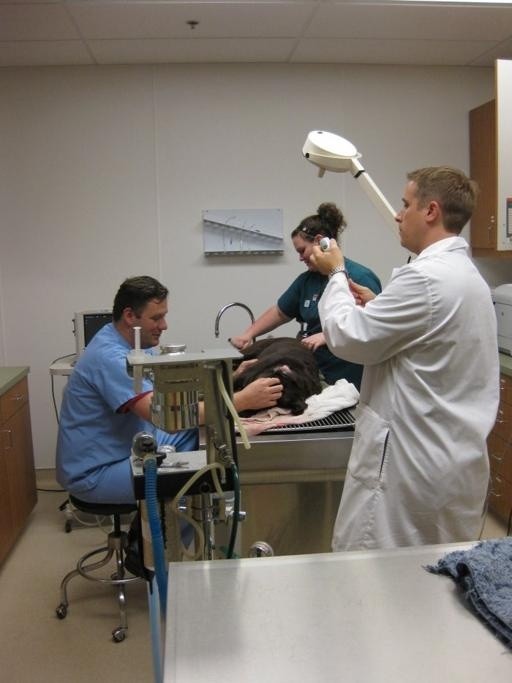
[309,166,500,553]
[56,275,284,556]
[230,202,382,393]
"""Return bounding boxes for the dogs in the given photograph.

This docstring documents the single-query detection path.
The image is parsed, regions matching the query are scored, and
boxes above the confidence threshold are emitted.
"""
[227,335,322,416]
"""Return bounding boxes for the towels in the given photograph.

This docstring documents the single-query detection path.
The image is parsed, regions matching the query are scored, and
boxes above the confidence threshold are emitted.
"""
[420,538,511,647]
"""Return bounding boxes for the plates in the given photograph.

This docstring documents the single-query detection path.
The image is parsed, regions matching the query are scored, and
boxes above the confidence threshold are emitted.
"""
[160,344,185,353]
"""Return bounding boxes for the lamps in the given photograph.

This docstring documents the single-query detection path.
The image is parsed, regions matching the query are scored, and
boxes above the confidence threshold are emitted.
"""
[300,127,416,263]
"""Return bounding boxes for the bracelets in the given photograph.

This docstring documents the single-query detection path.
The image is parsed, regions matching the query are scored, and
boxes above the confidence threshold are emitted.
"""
[328,264,347,281]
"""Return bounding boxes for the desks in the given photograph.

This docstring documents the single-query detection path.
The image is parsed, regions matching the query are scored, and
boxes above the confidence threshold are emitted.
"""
[164,538,511,682]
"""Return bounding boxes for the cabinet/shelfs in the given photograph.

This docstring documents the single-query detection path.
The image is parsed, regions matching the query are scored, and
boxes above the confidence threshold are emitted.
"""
[0,374,38,566]
[468,57,512,253]
[485,372,509,526]
